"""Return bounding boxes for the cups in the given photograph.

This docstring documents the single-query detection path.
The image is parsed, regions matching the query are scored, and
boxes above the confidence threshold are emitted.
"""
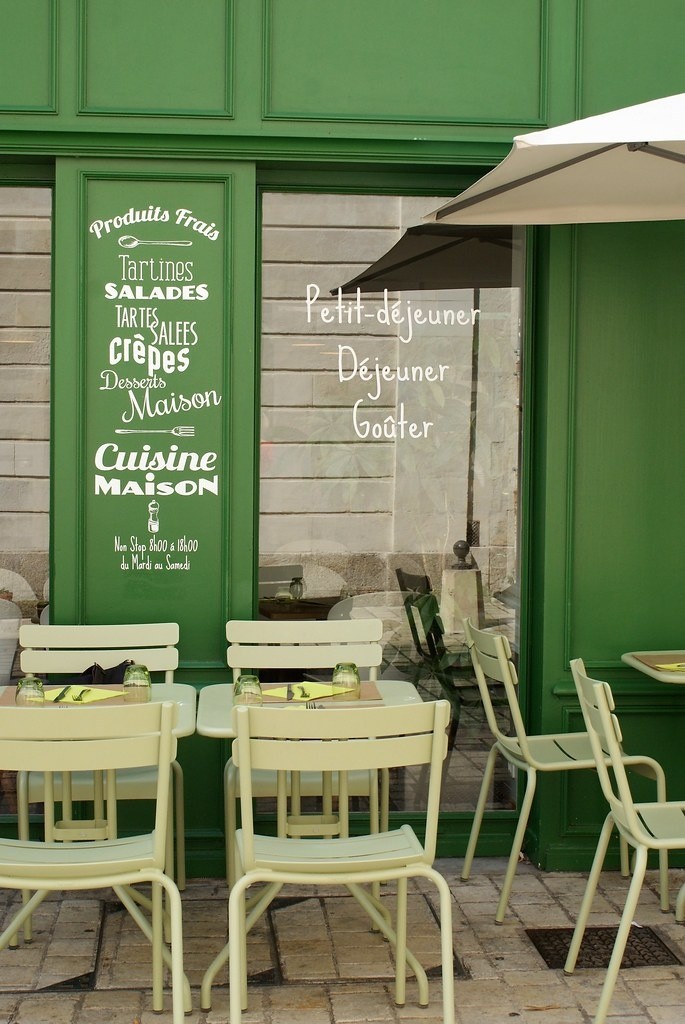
[232,675,263,709]
[124,664,151,702]
[15,677,46,709]
[332,662,360,701]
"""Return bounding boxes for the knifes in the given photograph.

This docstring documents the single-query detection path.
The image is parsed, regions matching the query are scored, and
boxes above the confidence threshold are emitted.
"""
[287,684,295,702]
[52,685,72,704]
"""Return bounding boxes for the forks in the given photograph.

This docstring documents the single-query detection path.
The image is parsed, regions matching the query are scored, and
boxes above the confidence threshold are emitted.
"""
[306,702,316,710]
[297,686,310,698]
[72,689,90,701]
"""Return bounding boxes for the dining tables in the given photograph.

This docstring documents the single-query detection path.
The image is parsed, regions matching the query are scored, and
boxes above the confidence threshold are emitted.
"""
[0,676,196,1015]
[195,681,431,1008]
[622,649,685,925]
[257,594,346,622]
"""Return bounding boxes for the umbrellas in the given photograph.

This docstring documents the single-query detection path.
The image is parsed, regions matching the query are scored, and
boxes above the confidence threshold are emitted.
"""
[413,90,685,227]
[336,224,514,293]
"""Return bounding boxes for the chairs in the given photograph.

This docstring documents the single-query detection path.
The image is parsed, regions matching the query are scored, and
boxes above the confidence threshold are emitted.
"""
[202,698,459,1024]
[396,567,518,776]
[564,658,685,1024]
[18,621,184,944]
[223,619,392,943]
[0,701,193,1024]
[458,617,670,923]
[405,589,512,812]
[0,566,51,686]
